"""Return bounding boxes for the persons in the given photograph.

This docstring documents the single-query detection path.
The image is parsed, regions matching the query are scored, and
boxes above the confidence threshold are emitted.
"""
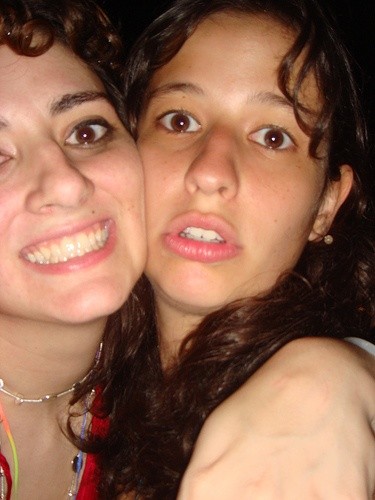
[60,1,375,500]
[1,0,375,499]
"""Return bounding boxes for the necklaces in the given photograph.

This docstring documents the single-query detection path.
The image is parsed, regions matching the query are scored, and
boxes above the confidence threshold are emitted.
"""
[0,339,105,405]
